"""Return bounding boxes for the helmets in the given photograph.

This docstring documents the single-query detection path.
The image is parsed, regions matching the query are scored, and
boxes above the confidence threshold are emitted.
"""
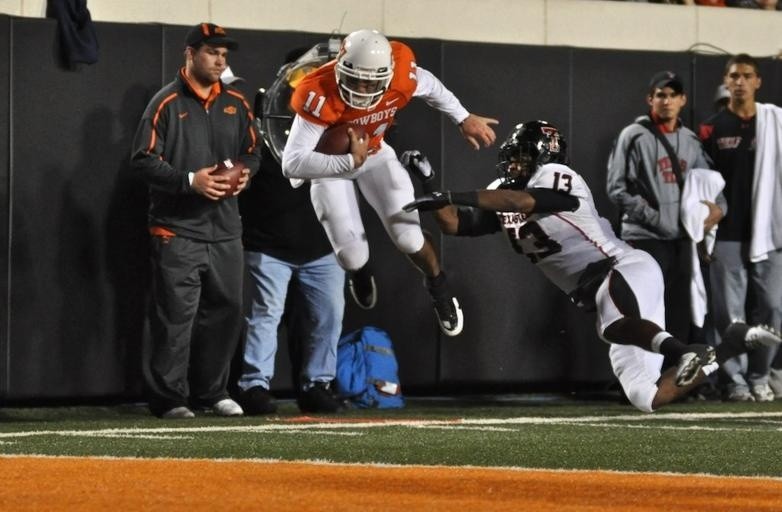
[495,119,567,187]
[334,28,395,113]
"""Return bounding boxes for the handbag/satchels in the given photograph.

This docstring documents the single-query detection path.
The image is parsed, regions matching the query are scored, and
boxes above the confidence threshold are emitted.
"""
[671,236,715,277]
[336,326,405,408]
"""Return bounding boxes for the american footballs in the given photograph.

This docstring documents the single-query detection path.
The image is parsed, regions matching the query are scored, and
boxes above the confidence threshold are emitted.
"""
[315,123,365,153]
[206,162,245,199]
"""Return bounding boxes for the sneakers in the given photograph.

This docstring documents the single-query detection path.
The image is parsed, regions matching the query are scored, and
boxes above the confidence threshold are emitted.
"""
[161,406,195,419]
[202,398,244,417]
[296,380,339,412]
[743,372,774,403]
[423,277,464,338]
[239,388,277,415]
[725,382,756,402]
[347,262,377,310]
[721,321,781,355]
[678,383,722,404]
[674,344,717,388]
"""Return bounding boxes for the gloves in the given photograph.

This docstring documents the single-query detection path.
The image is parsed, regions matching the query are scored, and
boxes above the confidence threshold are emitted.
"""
[402,190,479,213]
[400,150,440,195]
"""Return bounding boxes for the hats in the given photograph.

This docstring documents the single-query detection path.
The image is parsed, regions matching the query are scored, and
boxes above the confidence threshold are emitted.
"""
[714,84,731,103]
[185,23,239,51]
[647,71,683,95]
[220,66,245,87]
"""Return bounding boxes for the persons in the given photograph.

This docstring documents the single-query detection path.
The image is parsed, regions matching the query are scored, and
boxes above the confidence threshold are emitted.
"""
[234,164,348,413]
[130,23,262,417]
[711,84,729,111]
[606,71,729,349]
[699,54,782,402]
[284,30,500,338]
[402,117,782,413]
